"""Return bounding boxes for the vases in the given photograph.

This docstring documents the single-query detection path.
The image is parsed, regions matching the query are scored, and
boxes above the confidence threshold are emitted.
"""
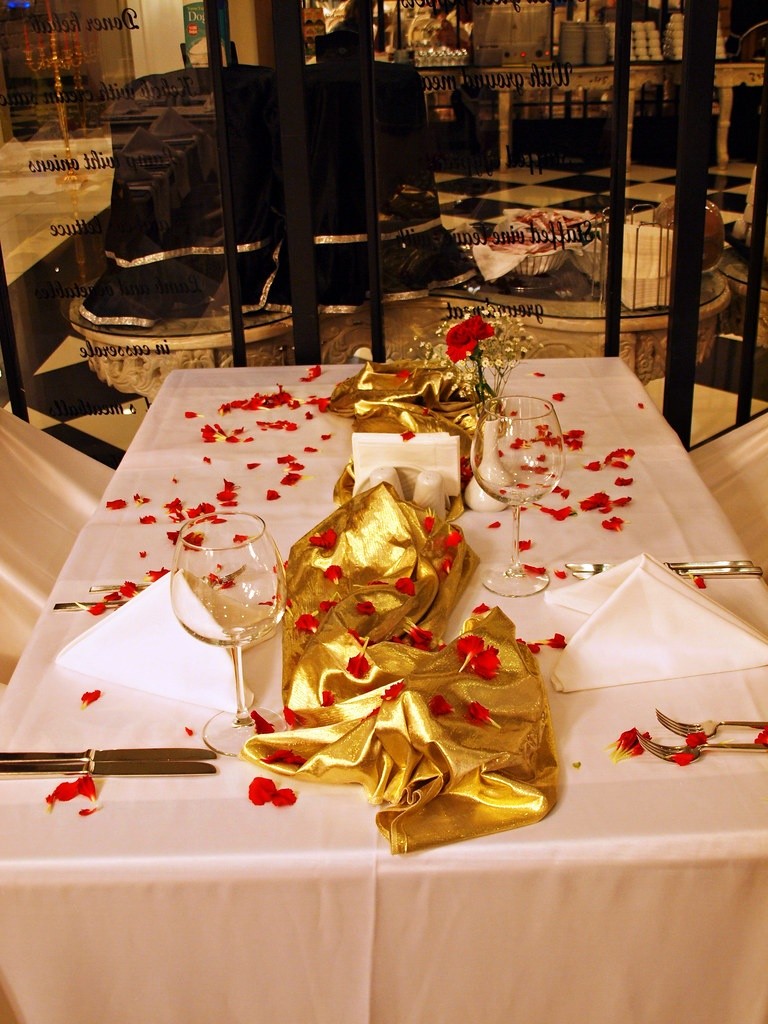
[464,419,515,513]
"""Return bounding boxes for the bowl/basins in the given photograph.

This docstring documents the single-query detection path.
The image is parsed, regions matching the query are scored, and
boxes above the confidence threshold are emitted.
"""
[665,13,727,59]
[606,22,663,60]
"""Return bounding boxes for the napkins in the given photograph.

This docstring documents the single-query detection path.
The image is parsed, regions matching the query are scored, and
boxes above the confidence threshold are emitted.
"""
[351,432,460,496]
[55,569,255,714]
[544,553,768,692]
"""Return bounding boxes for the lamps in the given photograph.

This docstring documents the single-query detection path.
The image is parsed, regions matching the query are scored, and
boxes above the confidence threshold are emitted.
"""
[22,0,87,184]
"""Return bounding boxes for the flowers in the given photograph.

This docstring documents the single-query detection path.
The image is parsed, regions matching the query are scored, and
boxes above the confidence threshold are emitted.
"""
[410,304,543,416]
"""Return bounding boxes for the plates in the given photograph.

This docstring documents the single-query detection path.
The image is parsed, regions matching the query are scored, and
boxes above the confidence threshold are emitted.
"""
[560,21,609,64]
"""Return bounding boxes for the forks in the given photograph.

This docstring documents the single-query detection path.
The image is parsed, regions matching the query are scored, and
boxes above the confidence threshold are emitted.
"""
[636,732,768,764]
[655,708,768,739]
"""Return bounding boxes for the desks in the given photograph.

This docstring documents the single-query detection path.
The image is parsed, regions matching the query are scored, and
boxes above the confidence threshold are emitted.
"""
[60,242,768,399]
[418,63,764,171]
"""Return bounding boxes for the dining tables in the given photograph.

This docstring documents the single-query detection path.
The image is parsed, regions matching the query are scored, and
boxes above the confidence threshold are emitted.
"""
[0,356,768,1024]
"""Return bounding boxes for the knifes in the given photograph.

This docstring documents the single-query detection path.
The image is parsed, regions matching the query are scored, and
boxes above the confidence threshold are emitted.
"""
[0,748,217,762]
[573,568,763,580]
[0,761,217,775]
[565,561,753,571]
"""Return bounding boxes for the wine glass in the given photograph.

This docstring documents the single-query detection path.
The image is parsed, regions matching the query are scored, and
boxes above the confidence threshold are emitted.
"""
[469,395,566,598]
[170,511,288,757]
